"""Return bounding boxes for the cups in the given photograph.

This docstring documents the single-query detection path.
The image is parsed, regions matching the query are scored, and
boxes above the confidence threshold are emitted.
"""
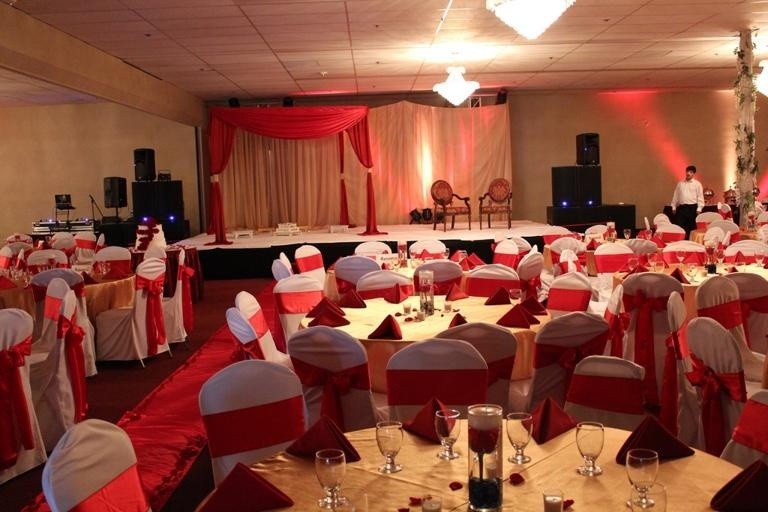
[629,482,667,512]
[420,494,443,512]
[623,228,663,243]
[397,268,451,323]
[542,488,565,512]
[466,402,504,512]
[335,487,369,512]
[509,289,522,307]
[2,257,111,288]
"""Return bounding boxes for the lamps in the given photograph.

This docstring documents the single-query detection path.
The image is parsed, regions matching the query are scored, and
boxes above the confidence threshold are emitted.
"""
[430,65,481,109]
[485,0,582,44]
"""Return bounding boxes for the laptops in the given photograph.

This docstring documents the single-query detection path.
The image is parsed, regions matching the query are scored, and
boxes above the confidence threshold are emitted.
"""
[55,194,75,210]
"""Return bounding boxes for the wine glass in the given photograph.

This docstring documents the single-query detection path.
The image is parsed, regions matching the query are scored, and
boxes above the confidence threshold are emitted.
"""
[506,411,534,463]
[626,448,661,508]
[385,237,468,270]
[376,420,404,473]
[314,447,348,510]
[435,409,462,460]
[575,421,605,476]
[626,245,766,285]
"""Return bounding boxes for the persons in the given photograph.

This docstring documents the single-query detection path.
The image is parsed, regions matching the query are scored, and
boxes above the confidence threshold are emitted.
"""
[669,165,706,233]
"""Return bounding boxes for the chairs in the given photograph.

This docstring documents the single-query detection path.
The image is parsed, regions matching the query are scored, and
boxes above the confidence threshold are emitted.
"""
[542,201,768,239]
[431,177,513,232]
[40,418,149,512]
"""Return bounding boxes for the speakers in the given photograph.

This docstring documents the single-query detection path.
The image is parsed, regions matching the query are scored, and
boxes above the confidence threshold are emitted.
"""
[576,133,600,165]
[134,148,155,180]
[132,180,183,225]
[577,166,601,207]
[552,166,578,207]
[104,176,127,208]
